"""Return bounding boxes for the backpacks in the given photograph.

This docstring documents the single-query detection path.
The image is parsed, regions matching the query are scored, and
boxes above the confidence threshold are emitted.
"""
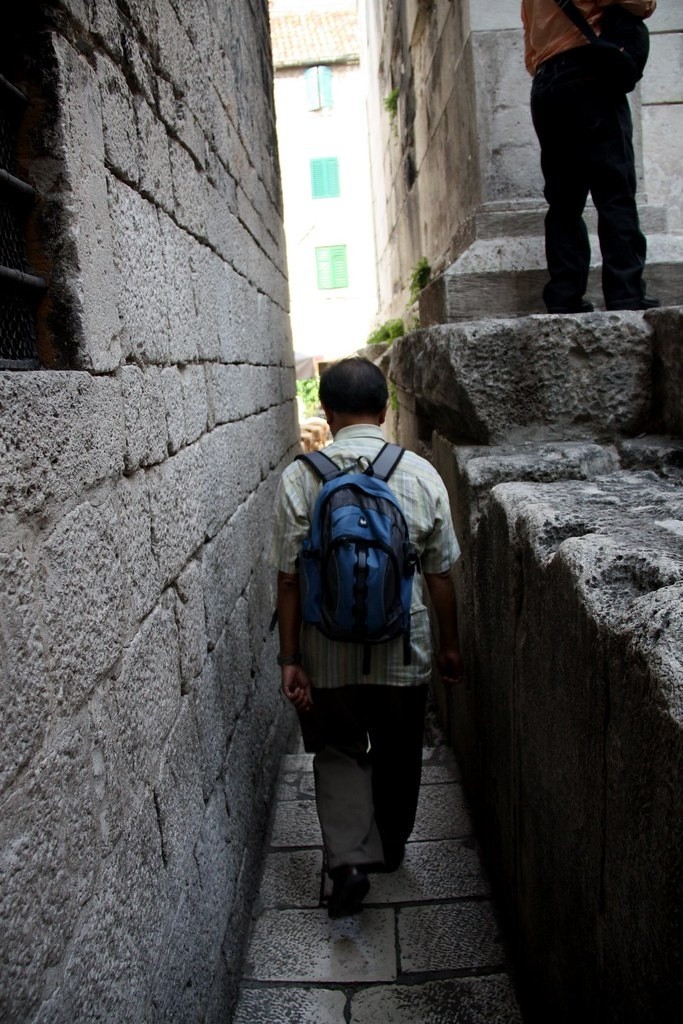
[295,442,417,648]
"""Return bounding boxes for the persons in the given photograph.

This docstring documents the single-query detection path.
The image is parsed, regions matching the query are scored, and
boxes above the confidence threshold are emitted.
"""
[264,358,464,917]
[522,0,662,314]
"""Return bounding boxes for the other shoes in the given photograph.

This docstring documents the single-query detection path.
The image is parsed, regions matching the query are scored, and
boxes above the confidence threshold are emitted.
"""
[547,299,594,314]
[375,846,405,874]
[614,294,661,308]
[327,866,370,920]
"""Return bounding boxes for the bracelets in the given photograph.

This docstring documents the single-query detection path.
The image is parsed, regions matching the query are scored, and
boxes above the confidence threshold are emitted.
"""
[277,653,303,664]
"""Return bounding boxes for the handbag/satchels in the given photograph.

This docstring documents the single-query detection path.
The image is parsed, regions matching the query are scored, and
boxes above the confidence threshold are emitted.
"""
[593,13,651,95]
